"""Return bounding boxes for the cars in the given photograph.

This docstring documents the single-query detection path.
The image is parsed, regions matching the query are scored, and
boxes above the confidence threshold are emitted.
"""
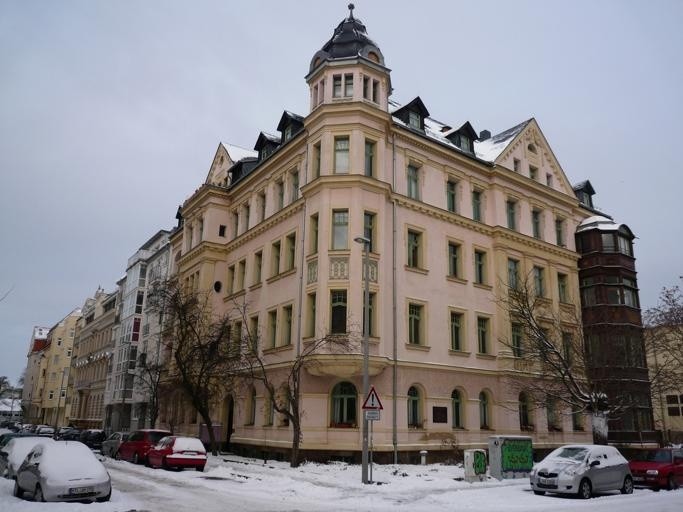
[525,442,637,501]
[11,436,114,504]
[143,435,208,474]
[1,416,174,481]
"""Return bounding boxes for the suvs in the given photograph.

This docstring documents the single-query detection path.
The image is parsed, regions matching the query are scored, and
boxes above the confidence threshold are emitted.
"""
[620,439,682,493]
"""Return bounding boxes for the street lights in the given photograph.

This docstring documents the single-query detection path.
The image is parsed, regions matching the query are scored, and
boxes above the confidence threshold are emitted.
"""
[116,340,132,431]
[352,234,372,486]
